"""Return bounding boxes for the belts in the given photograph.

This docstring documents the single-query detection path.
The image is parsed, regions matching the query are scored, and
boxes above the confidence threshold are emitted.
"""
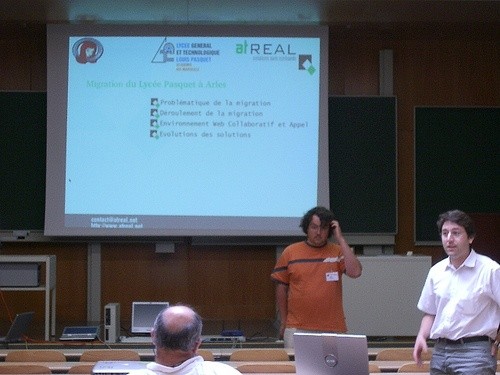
[438,336,489,345]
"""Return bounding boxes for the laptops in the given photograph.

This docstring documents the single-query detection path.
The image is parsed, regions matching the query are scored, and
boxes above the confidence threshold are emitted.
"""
[0,312,34,342]
[292,331,369,375]
[59,326,98,340]
[199,335,246,343]
[92,361,151,375]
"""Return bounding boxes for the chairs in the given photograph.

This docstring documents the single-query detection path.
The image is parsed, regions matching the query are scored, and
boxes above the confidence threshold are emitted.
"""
[0,350,500,375]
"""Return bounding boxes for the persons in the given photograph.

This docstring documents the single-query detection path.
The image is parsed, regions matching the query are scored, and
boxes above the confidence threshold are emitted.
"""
[412,209,499,375]
[269,206,362,361]
[123,304,245,375]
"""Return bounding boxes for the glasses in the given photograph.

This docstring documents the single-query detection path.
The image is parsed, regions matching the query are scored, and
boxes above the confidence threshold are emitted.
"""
[440,231,461,237]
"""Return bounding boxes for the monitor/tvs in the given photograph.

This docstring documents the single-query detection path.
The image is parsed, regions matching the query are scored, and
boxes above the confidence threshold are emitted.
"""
[131,302,169,337]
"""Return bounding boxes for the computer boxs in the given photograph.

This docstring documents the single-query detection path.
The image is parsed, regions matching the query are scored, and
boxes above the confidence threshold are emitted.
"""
[104,302,120,343]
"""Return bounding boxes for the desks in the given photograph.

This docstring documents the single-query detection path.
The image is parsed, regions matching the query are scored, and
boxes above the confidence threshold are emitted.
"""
[0,348,500,375]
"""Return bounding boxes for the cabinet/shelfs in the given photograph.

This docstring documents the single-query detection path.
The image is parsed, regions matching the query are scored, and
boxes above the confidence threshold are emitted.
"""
[0,254,56,342]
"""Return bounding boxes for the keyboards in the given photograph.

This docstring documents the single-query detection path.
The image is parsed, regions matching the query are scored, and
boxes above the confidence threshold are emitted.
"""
[121,336,152,343]
